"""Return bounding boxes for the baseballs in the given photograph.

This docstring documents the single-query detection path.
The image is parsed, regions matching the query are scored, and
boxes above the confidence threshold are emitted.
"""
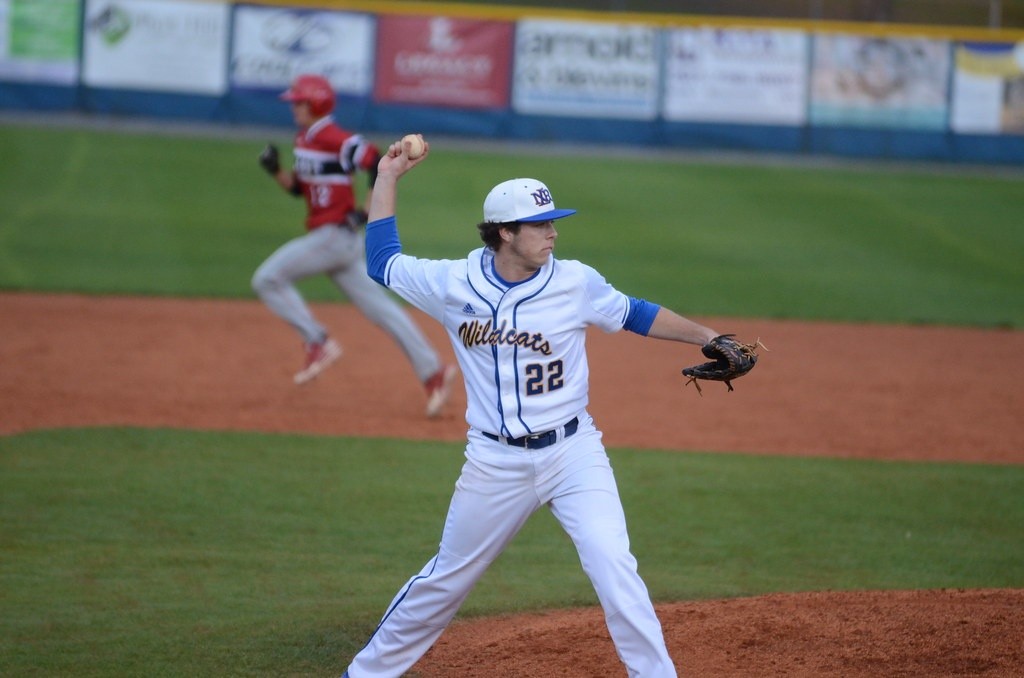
[401,135,426,160]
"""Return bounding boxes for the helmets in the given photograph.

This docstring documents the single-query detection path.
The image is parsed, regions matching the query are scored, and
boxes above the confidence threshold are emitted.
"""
[278,75,335,118]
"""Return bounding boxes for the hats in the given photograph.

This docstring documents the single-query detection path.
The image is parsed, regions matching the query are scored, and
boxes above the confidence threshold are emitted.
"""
[483,178,577,222]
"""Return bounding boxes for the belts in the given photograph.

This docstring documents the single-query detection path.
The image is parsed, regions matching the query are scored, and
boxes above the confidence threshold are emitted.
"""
[484,415,578,450]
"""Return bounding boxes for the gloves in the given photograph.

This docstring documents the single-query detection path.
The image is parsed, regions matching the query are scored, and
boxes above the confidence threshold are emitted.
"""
[257,145,279,174]
[340,208,368,230]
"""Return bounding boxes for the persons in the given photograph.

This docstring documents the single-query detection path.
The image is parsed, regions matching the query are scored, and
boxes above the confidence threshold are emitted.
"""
[251,74,455,419]
[343,135,759,678]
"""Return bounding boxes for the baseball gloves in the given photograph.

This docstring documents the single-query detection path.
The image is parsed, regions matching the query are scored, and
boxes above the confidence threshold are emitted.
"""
[681,337,758,384]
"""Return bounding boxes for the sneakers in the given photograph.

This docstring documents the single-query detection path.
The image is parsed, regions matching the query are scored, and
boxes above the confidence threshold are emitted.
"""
[294,339,341,384]
[423,366,455,418]
